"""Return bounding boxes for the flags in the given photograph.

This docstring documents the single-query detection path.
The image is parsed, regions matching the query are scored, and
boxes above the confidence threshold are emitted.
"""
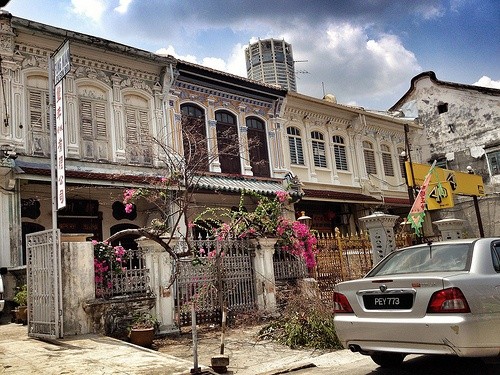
[406,168,433,236]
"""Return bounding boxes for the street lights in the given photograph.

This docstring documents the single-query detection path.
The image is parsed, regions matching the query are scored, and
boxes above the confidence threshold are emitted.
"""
[400,218,412,247]
[466,166,485,238]
[401,151,422,245]
[297,211,312,277]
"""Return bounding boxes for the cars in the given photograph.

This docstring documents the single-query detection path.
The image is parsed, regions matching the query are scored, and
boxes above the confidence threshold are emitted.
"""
[330,235,500,373]
